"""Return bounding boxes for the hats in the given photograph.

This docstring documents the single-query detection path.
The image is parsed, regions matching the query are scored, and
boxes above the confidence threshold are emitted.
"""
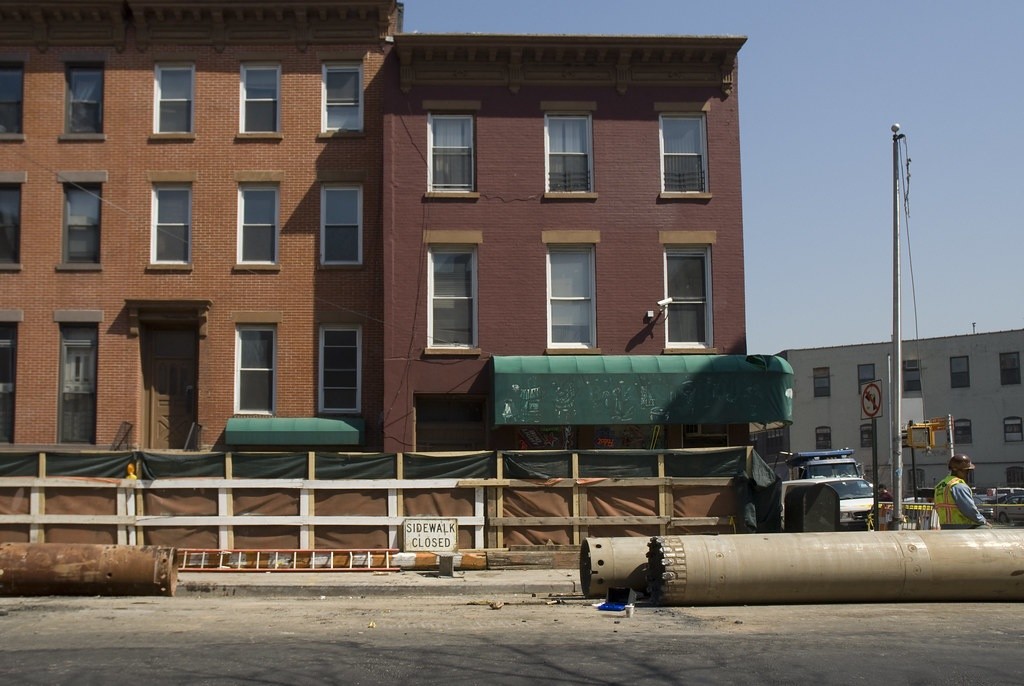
[878,484,886,489]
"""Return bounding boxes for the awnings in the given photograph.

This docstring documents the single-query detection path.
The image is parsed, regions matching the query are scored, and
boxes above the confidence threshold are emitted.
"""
[492,355,795,424]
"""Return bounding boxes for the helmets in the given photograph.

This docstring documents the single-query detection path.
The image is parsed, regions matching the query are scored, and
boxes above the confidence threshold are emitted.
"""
[948,454,976,470]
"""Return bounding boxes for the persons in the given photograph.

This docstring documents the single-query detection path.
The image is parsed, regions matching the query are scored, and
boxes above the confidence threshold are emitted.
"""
[935,454,992,529]
[225,418,364,445]
[878,484,894,502]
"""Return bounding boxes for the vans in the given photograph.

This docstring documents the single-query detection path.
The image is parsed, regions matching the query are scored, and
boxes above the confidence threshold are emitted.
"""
[987,487,1024,499]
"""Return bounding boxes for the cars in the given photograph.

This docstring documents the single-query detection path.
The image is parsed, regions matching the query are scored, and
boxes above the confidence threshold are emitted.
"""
[889,493,993,519]
[993,496,1024,525]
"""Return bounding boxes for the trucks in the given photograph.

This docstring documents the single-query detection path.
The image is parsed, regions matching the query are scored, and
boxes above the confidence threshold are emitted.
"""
[766,448,875,520]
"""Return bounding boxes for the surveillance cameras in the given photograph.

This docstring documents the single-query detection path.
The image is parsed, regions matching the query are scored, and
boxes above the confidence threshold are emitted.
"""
[657,298,673,306]
